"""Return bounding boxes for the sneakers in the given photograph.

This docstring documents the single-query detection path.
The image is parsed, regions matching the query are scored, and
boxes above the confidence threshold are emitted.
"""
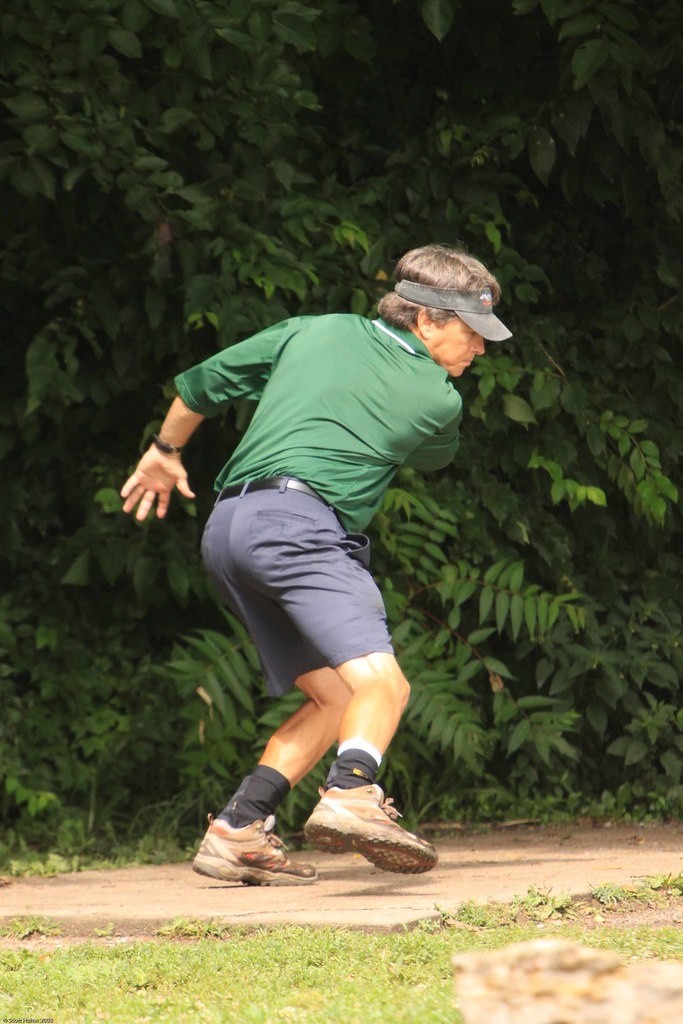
[192,813,317,888]
[304,784,438,875]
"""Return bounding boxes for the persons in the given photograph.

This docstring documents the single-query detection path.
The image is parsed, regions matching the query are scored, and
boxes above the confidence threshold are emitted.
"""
[120,244,515,884]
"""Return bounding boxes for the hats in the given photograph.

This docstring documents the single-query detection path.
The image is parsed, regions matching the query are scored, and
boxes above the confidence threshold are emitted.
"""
[395,280,513,341]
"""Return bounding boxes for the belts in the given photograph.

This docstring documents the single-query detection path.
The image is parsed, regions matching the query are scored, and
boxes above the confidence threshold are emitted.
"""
[220,477,330,506]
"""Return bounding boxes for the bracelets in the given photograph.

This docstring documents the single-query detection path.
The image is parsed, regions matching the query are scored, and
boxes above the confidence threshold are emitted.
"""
[153,435,185,453]
